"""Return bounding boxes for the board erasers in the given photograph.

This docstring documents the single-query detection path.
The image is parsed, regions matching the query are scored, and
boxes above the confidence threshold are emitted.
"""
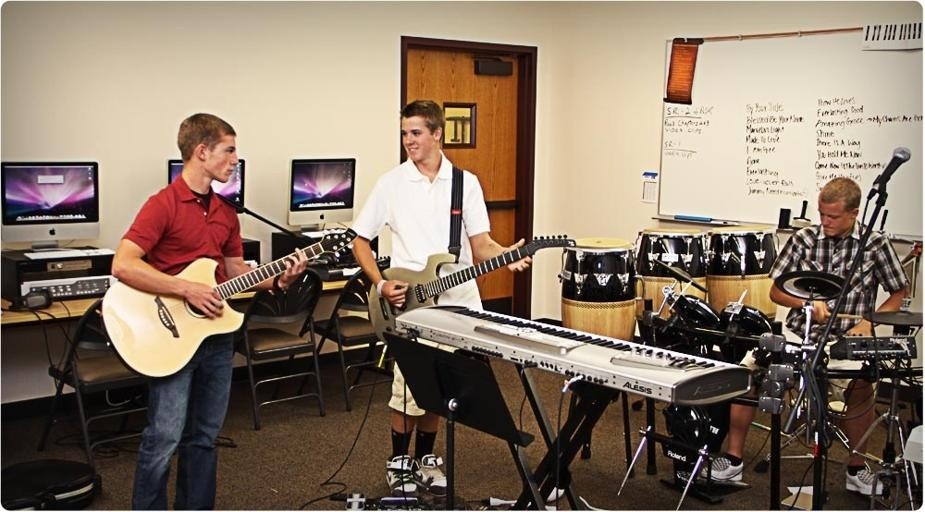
[778,208,791,232]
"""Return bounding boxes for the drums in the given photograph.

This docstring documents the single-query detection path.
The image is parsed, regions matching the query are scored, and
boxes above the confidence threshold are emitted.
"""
[703,231,779,333]
[638,234,710,347]
[557,243,638,341]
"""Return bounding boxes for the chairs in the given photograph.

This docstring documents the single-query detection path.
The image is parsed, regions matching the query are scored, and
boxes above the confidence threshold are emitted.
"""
[35,296,152,461]
[232,269,328,433]
[313,254,391,415]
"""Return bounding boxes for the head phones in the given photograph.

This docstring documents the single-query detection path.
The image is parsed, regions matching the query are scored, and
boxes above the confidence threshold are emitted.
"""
[9,290,54,311]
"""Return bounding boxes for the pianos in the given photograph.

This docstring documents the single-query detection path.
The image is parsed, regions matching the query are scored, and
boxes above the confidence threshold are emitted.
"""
[386,301,751,408]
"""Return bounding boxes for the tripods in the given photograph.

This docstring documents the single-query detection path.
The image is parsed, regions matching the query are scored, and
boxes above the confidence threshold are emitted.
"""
[820,328,924,511]
[754,306,873,476]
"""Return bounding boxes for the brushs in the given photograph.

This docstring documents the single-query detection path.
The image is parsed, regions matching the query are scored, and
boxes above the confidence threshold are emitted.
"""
[793,200,811,229]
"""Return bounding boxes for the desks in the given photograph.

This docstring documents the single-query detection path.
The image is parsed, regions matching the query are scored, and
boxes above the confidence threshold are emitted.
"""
[2,276,386,408]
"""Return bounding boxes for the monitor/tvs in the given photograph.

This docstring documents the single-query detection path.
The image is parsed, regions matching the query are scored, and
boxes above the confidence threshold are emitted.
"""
[169,159,244,213]
[0,161,99,252]
[289,160,354,238]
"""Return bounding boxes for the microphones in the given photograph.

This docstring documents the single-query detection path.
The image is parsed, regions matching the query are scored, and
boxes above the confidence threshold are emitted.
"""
[867,147,911,200]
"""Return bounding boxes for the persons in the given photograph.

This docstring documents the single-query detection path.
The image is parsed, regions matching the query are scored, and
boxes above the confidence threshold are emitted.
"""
[699,177,911,496]
[111,114,308,510]
[350,99,533,499]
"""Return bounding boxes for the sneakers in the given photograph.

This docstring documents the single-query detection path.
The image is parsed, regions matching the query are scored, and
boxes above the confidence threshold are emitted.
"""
[412,454,452,496]
[844,465,890,497]
[385,454,418,497]
[699,457,744,483]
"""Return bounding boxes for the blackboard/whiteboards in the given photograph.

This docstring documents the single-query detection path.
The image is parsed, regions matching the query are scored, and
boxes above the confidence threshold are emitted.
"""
[655,25,921,246]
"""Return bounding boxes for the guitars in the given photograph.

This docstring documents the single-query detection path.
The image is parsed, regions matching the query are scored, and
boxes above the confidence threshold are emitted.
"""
[102,226,356,381]
[367,235,580,346]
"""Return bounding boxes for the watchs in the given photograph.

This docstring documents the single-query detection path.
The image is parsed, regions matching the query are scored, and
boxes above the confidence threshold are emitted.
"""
[376,279,387,298]
[273,275,291,293]
